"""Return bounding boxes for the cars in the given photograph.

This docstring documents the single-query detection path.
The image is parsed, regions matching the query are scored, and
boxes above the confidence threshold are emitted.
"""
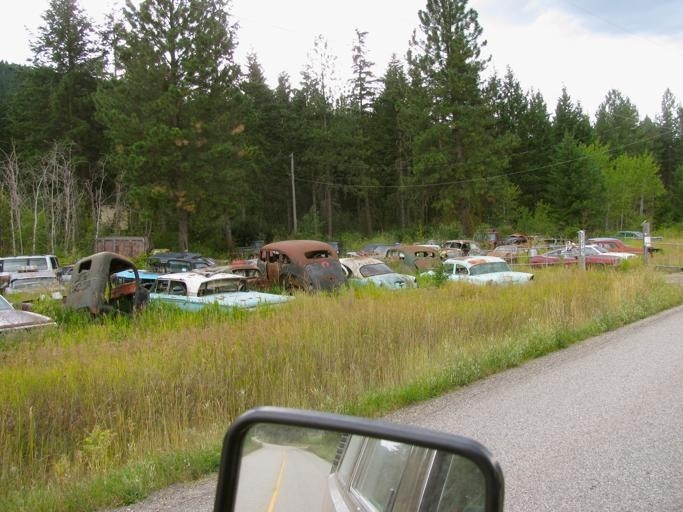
[322,434,490,512]
